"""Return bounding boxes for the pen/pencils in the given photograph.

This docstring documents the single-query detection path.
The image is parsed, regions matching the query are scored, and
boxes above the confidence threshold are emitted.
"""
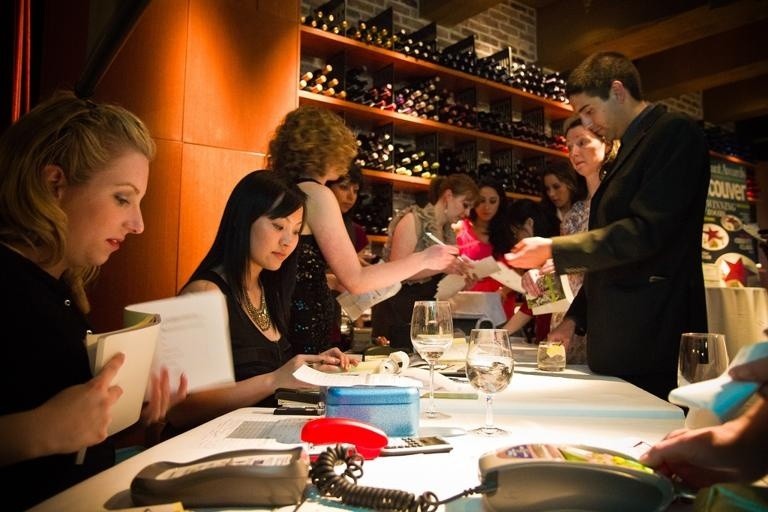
[424,232,465,264]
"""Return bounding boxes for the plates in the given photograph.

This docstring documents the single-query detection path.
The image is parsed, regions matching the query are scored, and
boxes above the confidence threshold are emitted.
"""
[721,214,744,232]
[701,224,730,252]
[715,252,760,288]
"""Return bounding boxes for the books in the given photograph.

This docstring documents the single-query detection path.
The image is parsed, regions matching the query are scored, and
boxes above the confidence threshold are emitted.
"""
[83,285,236,439]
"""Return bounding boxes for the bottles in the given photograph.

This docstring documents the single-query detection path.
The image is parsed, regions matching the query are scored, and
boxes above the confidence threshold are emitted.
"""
[300,9,571,239]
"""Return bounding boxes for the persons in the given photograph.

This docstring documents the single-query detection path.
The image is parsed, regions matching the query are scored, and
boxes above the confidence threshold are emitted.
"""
[643,359,767,496]
[1,98,185,509]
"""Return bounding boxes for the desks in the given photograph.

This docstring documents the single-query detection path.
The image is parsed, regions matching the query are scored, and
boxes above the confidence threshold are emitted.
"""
[321,350,690,418]
[701,286,766,363]
[30,405,701,512]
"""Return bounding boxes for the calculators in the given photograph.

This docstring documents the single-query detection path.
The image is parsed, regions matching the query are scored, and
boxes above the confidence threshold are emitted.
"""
[379,433,454,456]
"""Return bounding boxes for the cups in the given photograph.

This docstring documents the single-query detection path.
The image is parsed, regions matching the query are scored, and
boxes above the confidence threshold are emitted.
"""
[677,332,729,388]
[536,341,567,372]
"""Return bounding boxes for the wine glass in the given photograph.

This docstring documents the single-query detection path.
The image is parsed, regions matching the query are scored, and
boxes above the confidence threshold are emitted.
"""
[465,328,517,439]
[410,301,456,424]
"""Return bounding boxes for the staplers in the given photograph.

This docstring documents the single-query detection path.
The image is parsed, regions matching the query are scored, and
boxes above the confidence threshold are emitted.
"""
[301,418,388,463]
[273,388,326,416]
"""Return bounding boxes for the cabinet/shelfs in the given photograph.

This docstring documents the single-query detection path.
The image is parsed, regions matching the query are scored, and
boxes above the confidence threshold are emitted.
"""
[298,20,577,247]
